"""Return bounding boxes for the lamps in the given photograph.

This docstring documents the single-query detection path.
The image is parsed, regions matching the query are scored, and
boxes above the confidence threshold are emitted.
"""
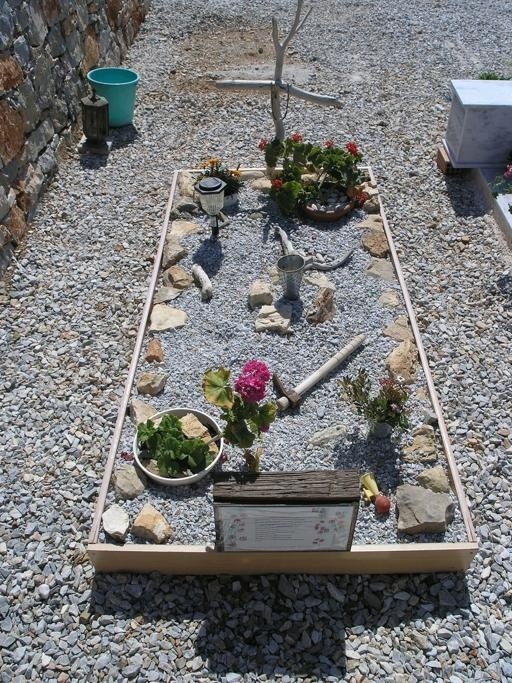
[194,176,228,235]
[80,86,114,155]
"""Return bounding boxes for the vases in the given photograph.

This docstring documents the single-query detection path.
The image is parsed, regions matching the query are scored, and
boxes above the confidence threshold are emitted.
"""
[133,407,224,486]
[223,192,237,206]
[368,418,393,438]
[299,181,355,223]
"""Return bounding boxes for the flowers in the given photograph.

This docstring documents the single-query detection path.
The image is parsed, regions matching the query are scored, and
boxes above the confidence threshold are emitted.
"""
[337,361,414,435]
[195,158,246,200]
[135,358,279,478]
[259,132,371,218]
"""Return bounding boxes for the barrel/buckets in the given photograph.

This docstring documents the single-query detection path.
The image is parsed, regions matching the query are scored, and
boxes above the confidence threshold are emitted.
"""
[87,67,140,127]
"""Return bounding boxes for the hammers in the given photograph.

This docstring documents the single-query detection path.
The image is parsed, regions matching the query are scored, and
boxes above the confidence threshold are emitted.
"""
[273,334,368,412]
